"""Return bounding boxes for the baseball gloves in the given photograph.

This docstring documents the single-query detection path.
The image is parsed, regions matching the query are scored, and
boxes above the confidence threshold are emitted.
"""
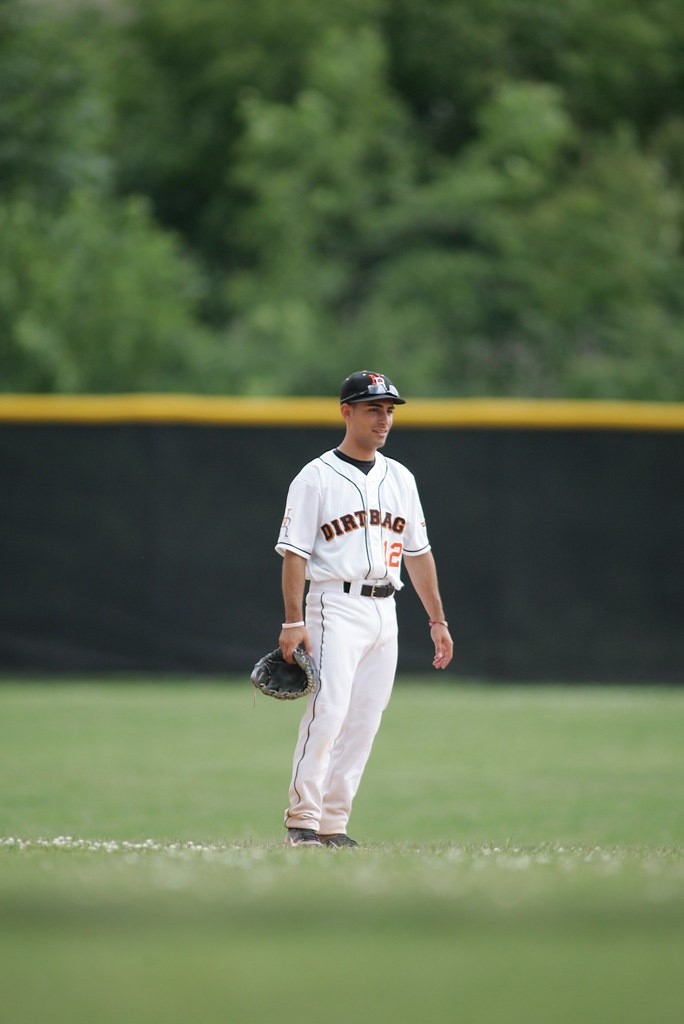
[249,645,315,700]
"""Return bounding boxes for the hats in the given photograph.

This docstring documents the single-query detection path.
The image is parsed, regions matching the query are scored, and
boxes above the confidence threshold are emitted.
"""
[339,370,406,405]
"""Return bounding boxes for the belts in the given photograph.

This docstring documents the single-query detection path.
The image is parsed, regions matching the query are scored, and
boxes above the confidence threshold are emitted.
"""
[344,581,395,599]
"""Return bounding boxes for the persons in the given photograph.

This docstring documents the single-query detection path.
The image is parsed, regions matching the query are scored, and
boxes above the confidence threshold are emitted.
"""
[251,370,453,851]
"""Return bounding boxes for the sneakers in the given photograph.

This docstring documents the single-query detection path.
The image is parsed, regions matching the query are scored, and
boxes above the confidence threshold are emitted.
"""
[283,828,325,849]
[317,834,361,851]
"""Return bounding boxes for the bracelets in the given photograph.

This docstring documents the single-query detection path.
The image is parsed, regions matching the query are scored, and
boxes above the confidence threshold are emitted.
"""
[282,621,305,628]
[429,620,448,627]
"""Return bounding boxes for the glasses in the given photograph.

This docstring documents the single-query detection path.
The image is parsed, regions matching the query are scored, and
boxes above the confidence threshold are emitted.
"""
[340,383,400,403]
